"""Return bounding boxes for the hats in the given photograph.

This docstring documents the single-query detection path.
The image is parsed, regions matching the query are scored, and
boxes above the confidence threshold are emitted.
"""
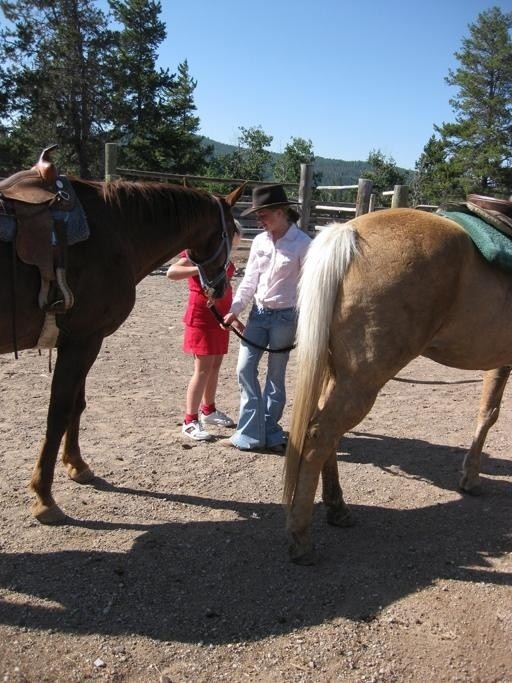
[240,184,302,216]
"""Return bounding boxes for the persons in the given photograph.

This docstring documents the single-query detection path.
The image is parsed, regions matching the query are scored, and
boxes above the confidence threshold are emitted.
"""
[220,183,313,452]
[166,218,242,441]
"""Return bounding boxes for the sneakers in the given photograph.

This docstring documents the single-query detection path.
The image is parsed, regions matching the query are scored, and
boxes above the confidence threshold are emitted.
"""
[182,419,211,440]
[201,410,234,426]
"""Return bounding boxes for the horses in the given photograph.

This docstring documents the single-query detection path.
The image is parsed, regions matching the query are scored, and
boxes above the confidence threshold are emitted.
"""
[0,176,250,525]
[282,208,512,566]
